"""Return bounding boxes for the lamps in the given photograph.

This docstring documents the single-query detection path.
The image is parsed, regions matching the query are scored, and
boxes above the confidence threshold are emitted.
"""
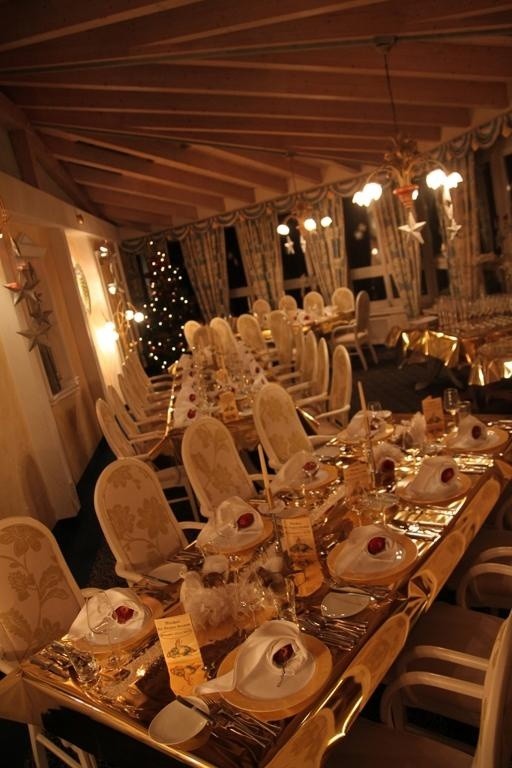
[276,150,333,255]
[351,35,464,247]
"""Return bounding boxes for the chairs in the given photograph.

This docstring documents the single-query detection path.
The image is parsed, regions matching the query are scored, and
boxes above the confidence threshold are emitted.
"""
[0,288,512,768]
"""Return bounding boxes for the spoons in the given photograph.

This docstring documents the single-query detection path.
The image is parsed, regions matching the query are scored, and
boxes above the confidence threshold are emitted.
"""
[298,606,369,652]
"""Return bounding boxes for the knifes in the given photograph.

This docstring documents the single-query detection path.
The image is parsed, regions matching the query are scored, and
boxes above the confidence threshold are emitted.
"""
[330,586,383,603]
[142,572,173,586]
[173,695,216,727]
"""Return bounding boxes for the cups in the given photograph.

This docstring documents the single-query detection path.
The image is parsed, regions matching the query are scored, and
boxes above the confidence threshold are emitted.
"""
[368,401,385,431]
[176,506,298,631]
[456,401,472,426]
[191,340,255,417]
[53,638,98,683]
[444,387,460,418]
[371,492,396,528]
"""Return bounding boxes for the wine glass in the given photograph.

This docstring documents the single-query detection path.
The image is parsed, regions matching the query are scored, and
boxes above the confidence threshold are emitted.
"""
[341,478,370,528]
[86,590,127,670]
[434,293,512,333]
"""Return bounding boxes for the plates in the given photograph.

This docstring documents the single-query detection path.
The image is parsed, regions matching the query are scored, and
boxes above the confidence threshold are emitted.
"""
[267,410,509,517]
[149,696,209,746]
[321,588,370,618]
[146,563,186,587]
[335,543,405,574]
[235,649,315,701]
[219,620,333,713]
[67,590,164,652]
[328,532,418,584]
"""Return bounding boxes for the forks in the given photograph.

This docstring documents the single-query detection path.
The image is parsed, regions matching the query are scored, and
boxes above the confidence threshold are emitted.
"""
[203,694,268,744]
